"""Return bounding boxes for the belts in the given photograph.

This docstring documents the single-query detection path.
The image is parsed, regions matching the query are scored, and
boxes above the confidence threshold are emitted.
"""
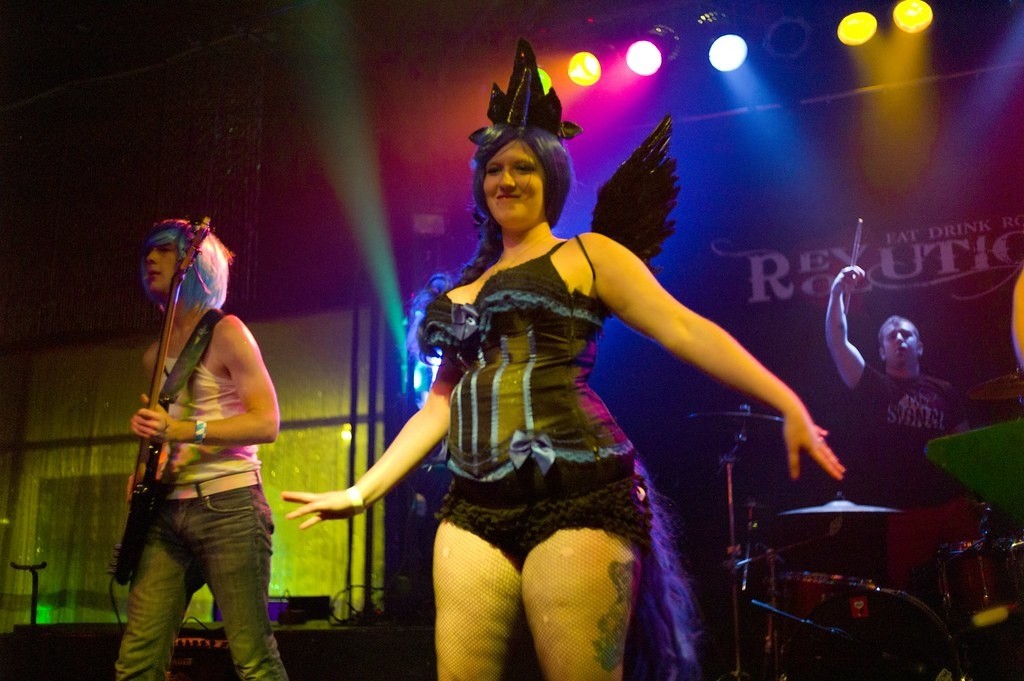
[166,470,263,501]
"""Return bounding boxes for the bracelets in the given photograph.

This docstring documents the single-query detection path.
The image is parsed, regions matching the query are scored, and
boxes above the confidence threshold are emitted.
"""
[194,420,207,445]
[347,488,363,515]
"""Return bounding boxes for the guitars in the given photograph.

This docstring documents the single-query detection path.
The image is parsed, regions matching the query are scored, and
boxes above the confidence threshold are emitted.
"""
[105,210,216,588]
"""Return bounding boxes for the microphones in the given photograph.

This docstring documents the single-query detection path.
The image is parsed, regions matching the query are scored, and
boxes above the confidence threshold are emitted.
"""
[909,664,929,678]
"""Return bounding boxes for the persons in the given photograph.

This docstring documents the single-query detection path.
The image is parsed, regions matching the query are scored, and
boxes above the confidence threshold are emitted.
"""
[110,218,290,680]
[824,263,974,495]
[282,124,847,681]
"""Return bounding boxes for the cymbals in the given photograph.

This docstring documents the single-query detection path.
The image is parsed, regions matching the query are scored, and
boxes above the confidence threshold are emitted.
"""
[778,495,906,518]
[967,364,1023,400]
[686,401,783,436]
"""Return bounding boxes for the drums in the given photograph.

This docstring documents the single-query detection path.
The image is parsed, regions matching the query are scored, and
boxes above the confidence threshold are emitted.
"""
[933,536,1023,619]
[779,587,962,681]
[775,569,875,657]
